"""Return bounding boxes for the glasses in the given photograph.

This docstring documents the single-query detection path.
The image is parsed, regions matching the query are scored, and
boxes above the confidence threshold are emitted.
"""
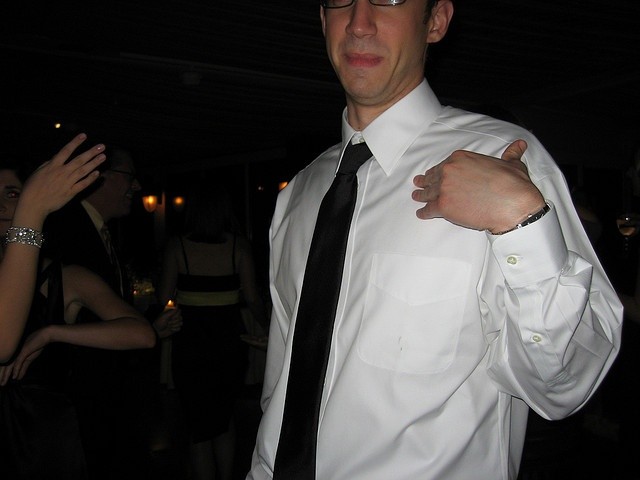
[316,0,405,9]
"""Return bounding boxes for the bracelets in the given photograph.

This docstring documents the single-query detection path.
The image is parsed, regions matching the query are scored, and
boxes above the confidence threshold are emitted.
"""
[3,226,46,249]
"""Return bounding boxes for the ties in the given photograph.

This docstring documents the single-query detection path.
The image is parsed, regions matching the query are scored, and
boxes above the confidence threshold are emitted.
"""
[269,144,375,479]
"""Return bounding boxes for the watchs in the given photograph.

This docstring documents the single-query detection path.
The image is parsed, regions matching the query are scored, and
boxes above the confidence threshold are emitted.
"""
[492,205,550,235]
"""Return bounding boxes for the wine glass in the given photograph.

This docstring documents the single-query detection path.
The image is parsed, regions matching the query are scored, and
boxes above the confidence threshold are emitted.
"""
[615,208,640,264]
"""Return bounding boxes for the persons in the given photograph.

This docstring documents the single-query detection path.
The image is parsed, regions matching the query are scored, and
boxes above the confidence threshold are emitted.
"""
[243,0,624,480]
[1,132,158,479]
[43,140,184,479]
[153,174,258,479]
